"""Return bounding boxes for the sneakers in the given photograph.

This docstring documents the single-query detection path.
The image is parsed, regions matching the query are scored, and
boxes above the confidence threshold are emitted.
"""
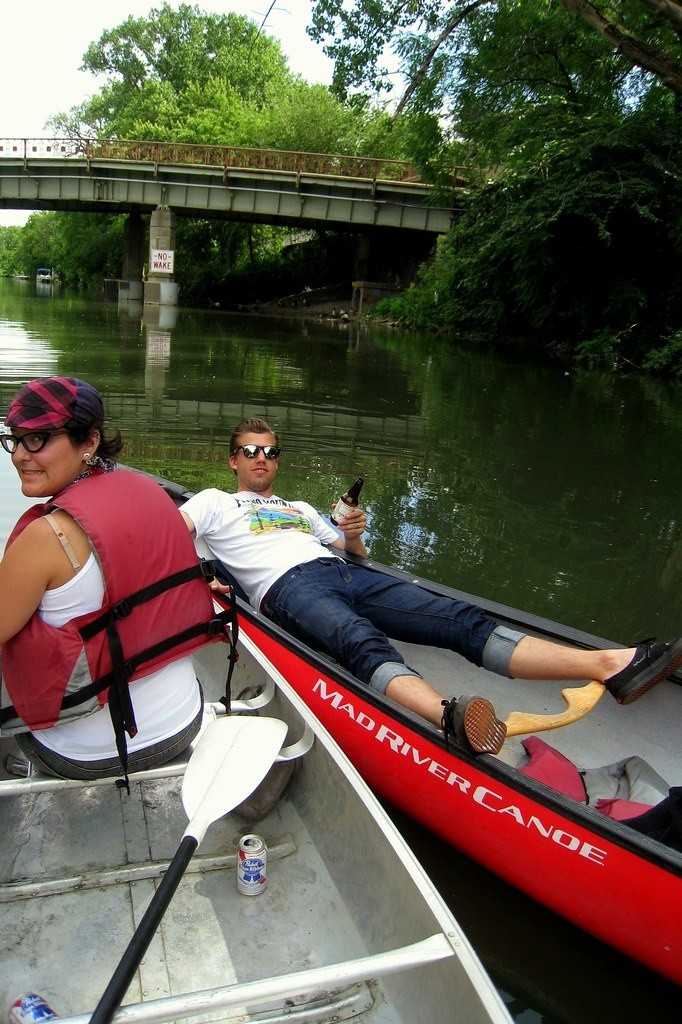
[602,635,682,705]
[441,696,508,755]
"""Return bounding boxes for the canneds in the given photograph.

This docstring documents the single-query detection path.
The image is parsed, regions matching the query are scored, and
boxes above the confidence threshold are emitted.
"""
[236,834,269,898]
[11,993,59,1024]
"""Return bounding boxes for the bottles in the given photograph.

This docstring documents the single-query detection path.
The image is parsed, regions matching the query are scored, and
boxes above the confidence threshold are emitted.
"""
[330,477,364,528]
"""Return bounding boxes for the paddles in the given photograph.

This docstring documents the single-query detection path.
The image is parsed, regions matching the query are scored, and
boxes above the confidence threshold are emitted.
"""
[88,716,290,1024]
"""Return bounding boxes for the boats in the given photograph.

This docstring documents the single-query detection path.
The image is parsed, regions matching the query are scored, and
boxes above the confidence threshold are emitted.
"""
[0,597,528,1024]
[15,275,30,279]
[108,461,682,995]
[36,268,51,282]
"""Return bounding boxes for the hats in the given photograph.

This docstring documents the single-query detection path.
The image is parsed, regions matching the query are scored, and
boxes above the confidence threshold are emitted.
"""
[5,376,104,431]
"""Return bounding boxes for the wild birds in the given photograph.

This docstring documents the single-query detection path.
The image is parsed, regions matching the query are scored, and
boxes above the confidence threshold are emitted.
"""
[331,305,355,323]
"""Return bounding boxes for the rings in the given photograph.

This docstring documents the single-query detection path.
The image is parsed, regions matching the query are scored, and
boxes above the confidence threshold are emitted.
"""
[357,523,359,528]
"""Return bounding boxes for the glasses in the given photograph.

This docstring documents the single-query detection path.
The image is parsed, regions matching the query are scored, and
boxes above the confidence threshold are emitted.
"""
[230,444,281,459]
[0,425,97,453]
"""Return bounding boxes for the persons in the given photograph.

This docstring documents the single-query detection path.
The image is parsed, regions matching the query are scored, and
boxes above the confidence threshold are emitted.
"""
[179,418,682,753]
[0,377,238,781]
